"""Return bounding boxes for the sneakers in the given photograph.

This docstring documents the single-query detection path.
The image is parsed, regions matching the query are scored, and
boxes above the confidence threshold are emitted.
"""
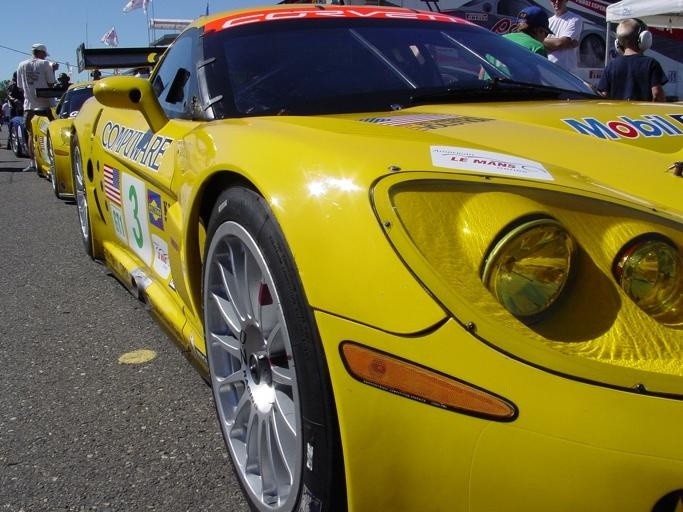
[22,166,36,172]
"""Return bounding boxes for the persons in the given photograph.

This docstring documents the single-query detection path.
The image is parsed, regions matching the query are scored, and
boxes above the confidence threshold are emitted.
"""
[596,18,669,102]
[479,0,583,80]
[0,44,74,172]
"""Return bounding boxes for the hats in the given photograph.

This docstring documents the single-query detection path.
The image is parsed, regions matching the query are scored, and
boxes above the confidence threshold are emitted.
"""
[12,72,17,82]
[57,73,70,81]
[32,44,50,56]
[516,7,553,34]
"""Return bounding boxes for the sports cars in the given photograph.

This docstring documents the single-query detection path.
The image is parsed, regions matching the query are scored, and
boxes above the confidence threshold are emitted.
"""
[8,79,100,200]
[69,2,682,512]
[437,0,555,42]
